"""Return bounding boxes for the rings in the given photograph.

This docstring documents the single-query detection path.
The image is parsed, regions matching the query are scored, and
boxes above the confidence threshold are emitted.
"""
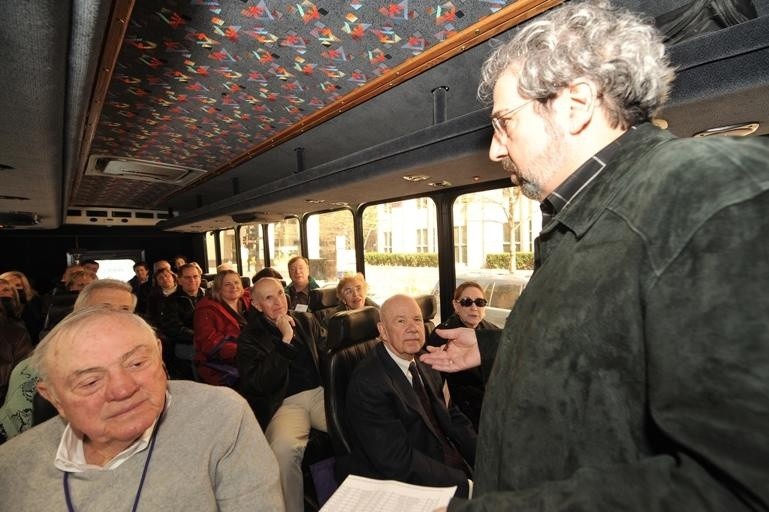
[448,359,454,365]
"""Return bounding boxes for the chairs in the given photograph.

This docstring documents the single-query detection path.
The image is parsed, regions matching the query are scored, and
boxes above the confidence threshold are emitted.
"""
[0,279,81,425]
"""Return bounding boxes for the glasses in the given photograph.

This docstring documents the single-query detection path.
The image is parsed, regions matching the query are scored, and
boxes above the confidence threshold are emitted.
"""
[455,297,487,306]
[492,98,544,143]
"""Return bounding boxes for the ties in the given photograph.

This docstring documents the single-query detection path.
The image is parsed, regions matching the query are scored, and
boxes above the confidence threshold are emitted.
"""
[410,364,446,442]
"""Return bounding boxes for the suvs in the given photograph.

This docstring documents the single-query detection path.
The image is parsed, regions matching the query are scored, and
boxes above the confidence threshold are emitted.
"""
[425,273,531,330]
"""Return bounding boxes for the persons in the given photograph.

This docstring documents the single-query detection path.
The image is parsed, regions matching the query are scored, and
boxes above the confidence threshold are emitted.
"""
[420,280,503,433]
[235,276,334,512]
[342,292,479,500]
[0,278,138,444]
[0,305,286,512]
[1,256,382,404]
[417,0,769,512]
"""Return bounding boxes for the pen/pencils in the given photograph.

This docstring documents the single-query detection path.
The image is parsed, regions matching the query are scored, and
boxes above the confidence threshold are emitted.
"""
[443,339,451,350]
